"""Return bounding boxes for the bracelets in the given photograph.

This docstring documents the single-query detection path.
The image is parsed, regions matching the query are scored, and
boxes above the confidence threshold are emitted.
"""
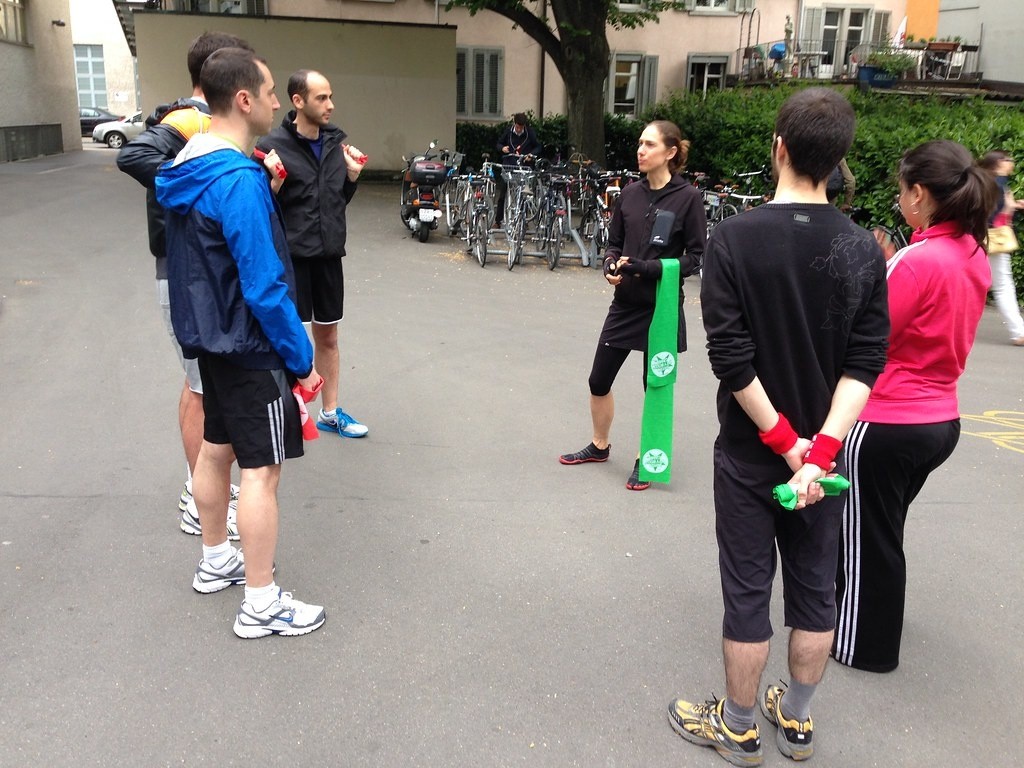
[803,433,843,471]
[759,412,798,456]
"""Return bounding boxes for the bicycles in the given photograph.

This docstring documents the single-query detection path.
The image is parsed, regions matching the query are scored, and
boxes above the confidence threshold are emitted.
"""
[447,146,910,274]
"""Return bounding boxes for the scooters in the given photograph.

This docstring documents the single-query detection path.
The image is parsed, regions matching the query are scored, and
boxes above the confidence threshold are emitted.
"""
[400,138,448,243]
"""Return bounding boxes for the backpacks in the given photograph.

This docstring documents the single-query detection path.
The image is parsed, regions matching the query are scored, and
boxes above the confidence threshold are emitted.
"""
[826,166,843,202]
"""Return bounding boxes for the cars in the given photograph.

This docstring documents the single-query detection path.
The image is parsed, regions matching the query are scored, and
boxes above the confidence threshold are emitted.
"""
[92,110,144,149]
[78,106,126,137]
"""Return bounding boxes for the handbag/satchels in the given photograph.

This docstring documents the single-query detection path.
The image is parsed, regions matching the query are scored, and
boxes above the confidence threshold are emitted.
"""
[984,226,1019,254]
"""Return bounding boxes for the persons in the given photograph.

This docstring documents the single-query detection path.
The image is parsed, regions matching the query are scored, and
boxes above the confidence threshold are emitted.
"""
[828,157,856,212]
[557,122,707,491]
[831,139,1002,675]
[661,87,891,768]
[491,114,541,230]
[249,68,370,436]
[975,148,1023,346]
[151,47,328,639]
[782,13,793,60]
[116,29,256,541]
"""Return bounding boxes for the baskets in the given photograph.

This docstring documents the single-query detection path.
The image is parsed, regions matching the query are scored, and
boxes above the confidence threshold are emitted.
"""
[501,165,533,184]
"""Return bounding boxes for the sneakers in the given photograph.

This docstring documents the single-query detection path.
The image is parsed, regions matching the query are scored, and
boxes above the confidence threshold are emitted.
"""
[233,586,326,639]
[178,480,240,510]
[559,442,611,464]
[626,458,652,491]
[760,678,814,761]
[316,408,369,439]
[180,499,240,540]
[667,692,763,767]
[193,546,276,593]
